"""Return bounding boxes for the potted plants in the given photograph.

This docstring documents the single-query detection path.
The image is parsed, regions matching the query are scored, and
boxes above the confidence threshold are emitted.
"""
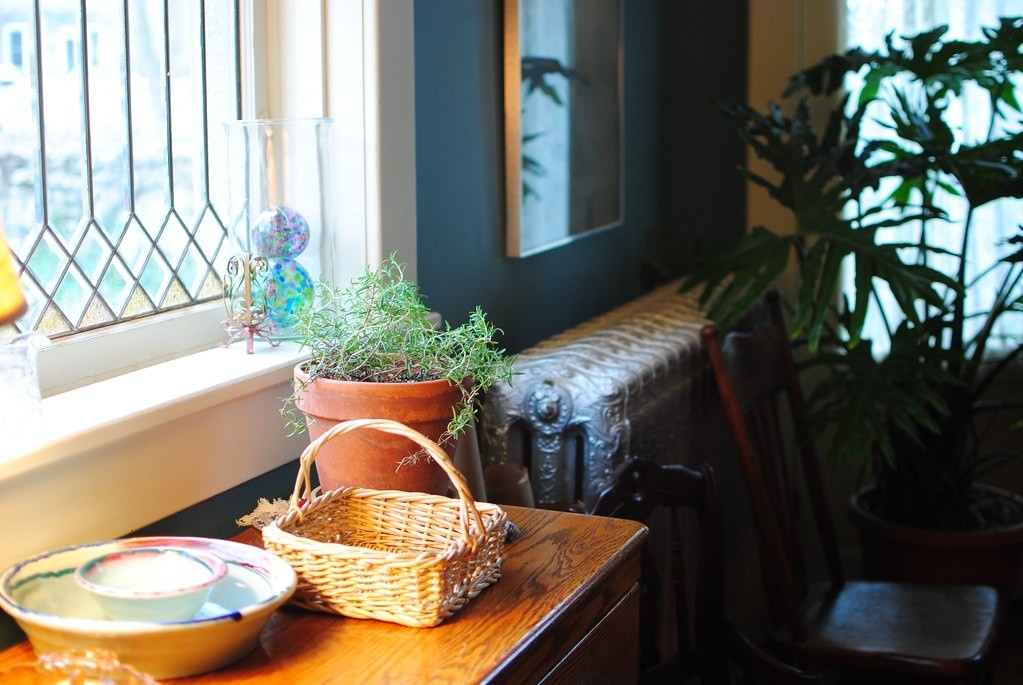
[672,17,1023,685]
[276,249,527,503]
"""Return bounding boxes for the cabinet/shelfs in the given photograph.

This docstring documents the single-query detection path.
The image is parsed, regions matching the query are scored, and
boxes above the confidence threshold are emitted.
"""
[0,503,650,685]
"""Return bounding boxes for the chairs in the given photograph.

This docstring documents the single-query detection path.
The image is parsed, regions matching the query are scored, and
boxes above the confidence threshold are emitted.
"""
[700,288,1001,685]
[592,457,820,685]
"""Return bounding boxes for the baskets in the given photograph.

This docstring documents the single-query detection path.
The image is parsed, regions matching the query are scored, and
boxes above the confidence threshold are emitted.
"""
[262,419,508,629]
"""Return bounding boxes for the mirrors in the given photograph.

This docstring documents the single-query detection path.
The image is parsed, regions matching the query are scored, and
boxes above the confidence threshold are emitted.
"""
[503,0,625,259]
